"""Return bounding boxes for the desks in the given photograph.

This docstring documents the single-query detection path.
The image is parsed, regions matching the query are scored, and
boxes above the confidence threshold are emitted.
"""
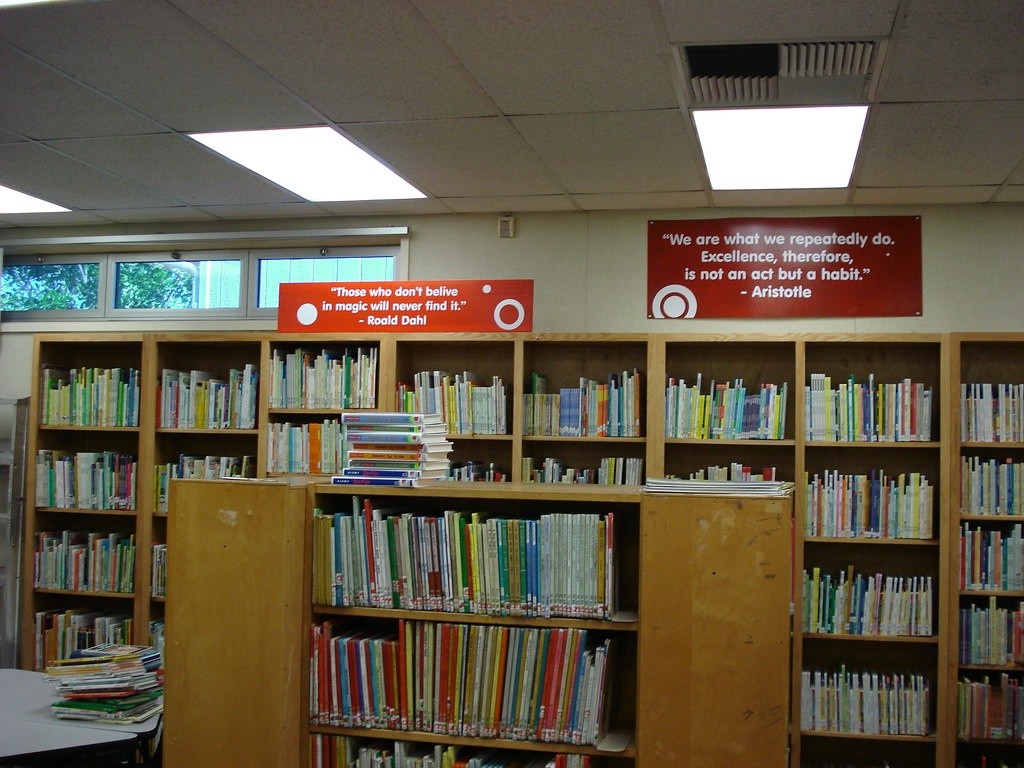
[0,667,162,766]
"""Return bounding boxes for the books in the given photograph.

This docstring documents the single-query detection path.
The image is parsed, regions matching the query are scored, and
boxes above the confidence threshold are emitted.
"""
[330,412,454,488]
[956,382,1024,742]
[44,643,164,726]
[799,564,934,637]
[800,664,929,735]
[267,344,378,478]
[304,496,621,767]
[803,372,935,443]
[395,369,511,483]
[34,363,260,672]
[646,364,794,502]
[803,467,932,542]
[521,368,644,485]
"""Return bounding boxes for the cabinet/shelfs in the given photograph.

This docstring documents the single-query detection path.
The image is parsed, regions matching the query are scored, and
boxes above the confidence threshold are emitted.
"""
[264,334,387,479]
[658,332,948,766]
[389,333,656,485]
[162,479,788,767]
[20,332,261,692]
[949,331,1024,767]
